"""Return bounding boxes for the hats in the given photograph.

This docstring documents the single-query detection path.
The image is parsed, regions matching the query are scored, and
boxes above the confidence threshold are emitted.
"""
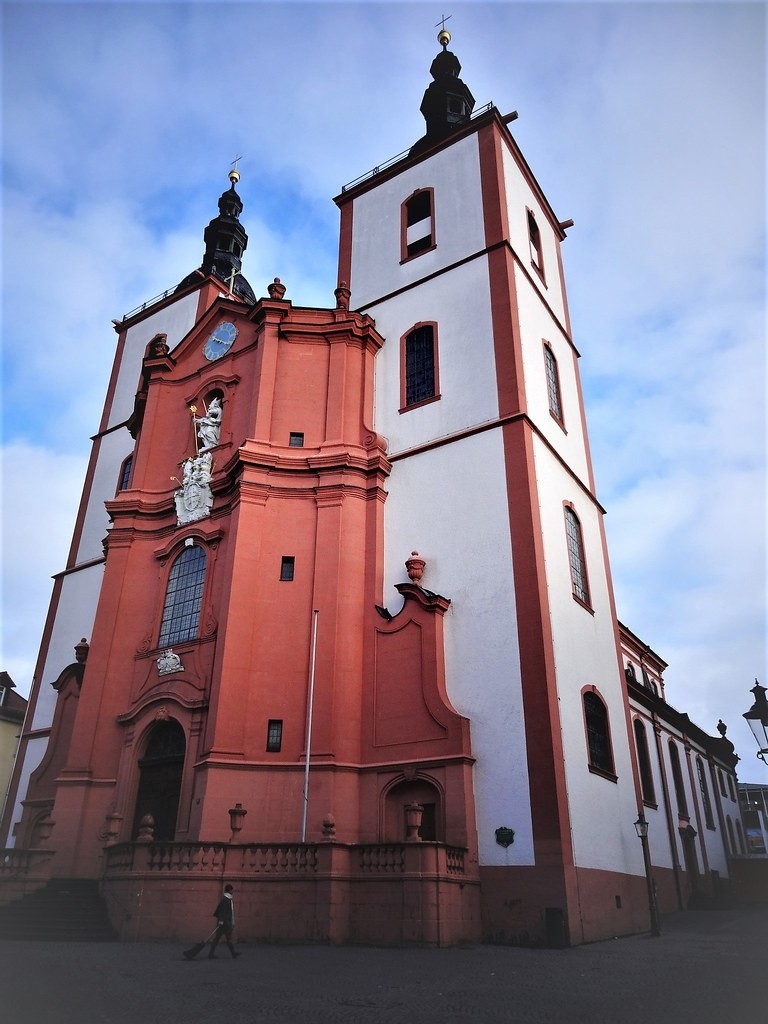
[224,884,233,891]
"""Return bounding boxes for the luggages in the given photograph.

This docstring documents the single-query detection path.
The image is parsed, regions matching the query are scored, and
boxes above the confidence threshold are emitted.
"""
[182,925,220,960]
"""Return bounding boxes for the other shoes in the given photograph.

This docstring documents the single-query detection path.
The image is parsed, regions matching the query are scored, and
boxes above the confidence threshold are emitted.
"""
[208,952,219,959]
[231,951,242,959]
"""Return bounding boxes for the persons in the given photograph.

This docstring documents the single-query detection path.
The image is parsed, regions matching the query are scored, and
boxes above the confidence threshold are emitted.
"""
[208,884,242,959]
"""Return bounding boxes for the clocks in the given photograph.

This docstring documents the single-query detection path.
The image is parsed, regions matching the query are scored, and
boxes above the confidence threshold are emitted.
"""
[204,322,238,361]
